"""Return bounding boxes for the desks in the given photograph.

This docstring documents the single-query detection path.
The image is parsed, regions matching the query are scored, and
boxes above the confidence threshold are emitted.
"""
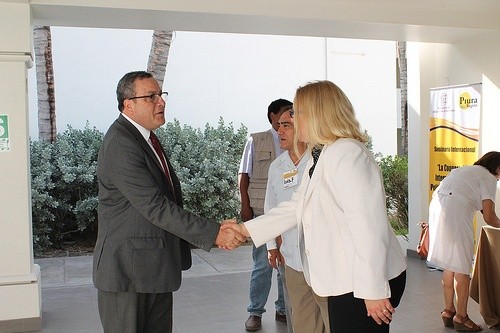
[468,224,500,329]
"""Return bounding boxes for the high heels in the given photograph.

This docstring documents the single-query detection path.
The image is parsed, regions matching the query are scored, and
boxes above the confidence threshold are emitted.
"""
[441,309,456,327]
[453,313,482,331]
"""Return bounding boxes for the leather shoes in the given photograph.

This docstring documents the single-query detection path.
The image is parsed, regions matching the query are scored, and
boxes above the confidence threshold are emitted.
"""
[245,315,261,330]
[276,311,286,322]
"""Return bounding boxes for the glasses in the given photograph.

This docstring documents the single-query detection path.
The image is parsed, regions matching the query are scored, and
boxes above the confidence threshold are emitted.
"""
[128,92,169,103]
[289,111,294,118]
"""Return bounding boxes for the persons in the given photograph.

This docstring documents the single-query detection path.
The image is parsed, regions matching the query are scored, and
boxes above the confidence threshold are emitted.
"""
[217,79,409,333]
[91,70,247,332]
[263,104,331,333]
[425,150,500,332]
[238,98,295,331]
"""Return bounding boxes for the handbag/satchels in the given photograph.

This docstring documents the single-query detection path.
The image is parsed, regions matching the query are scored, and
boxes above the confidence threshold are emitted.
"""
[416,221,430,256]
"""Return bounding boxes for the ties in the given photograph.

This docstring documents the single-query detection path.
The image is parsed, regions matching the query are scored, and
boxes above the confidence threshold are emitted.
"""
[149,131,175,199]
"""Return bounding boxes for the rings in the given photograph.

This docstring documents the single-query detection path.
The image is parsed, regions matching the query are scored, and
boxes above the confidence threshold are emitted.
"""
[382,309,388,313]
